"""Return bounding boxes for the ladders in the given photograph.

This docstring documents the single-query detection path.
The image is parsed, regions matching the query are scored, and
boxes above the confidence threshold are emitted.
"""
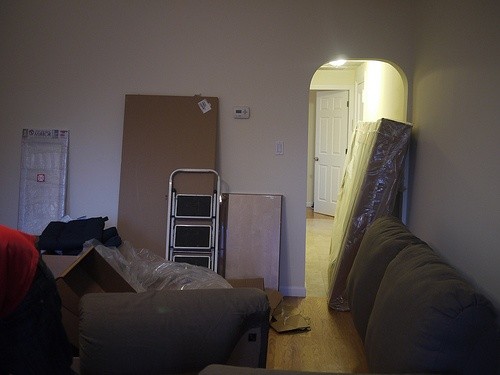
[165,169,220,274]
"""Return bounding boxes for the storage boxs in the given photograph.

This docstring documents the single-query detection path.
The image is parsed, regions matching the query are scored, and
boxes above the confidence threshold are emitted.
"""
[55,246,137,319]
